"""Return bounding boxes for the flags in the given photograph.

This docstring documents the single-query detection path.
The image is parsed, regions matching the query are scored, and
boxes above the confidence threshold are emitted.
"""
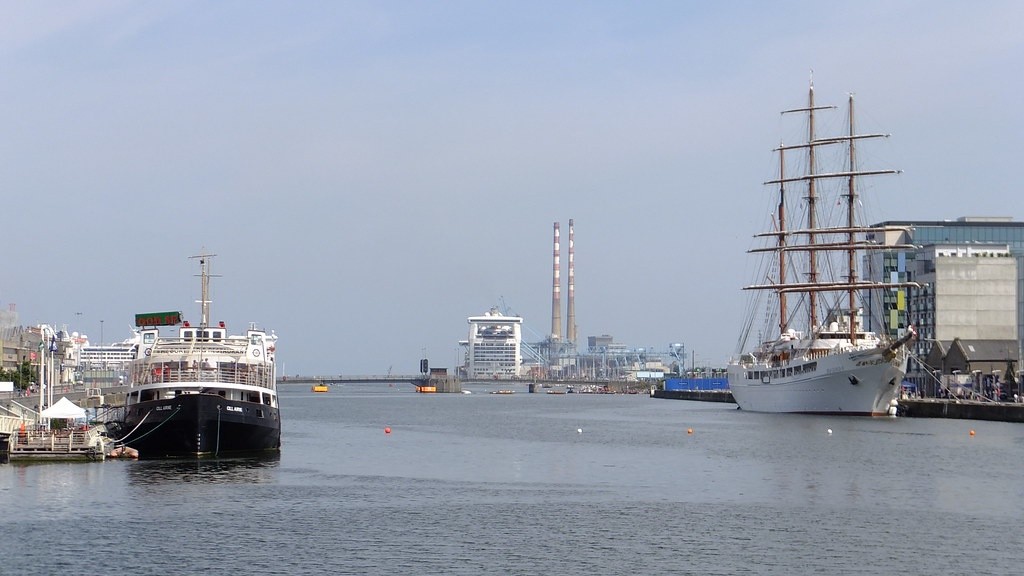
[50,336,58,351]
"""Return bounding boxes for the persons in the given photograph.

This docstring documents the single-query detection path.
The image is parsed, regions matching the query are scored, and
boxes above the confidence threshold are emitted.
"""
[909,390,912,396]
[192,358,210,368]
[164,364,170,373]
[988,385,1000,400]
[944,386,950,398]
[920,389,924,398]
[962,389,979,400]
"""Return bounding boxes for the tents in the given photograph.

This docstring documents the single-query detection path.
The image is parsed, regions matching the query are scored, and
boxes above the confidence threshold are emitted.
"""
[900,381,917,398]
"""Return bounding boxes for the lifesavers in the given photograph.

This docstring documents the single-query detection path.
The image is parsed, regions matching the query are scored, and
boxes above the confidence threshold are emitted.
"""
[144,348,152,357]
[253,349,261,357]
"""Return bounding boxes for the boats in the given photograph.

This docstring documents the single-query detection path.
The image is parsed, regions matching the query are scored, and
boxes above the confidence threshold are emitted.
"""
[103,247,283,461]
[491,389,516,394]
[312,385,328,392]
[547,387,638,394]
[111,446,139,458]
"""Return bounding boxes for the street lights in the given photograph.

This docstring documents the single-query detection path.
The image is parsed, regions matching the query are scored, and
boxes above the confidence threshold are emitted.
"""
[17,362,24,396]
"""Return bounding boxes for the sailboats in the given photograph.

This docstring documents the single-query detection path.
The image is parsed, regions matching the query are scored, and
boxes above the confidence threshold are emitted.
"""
[726,67,930,413]
